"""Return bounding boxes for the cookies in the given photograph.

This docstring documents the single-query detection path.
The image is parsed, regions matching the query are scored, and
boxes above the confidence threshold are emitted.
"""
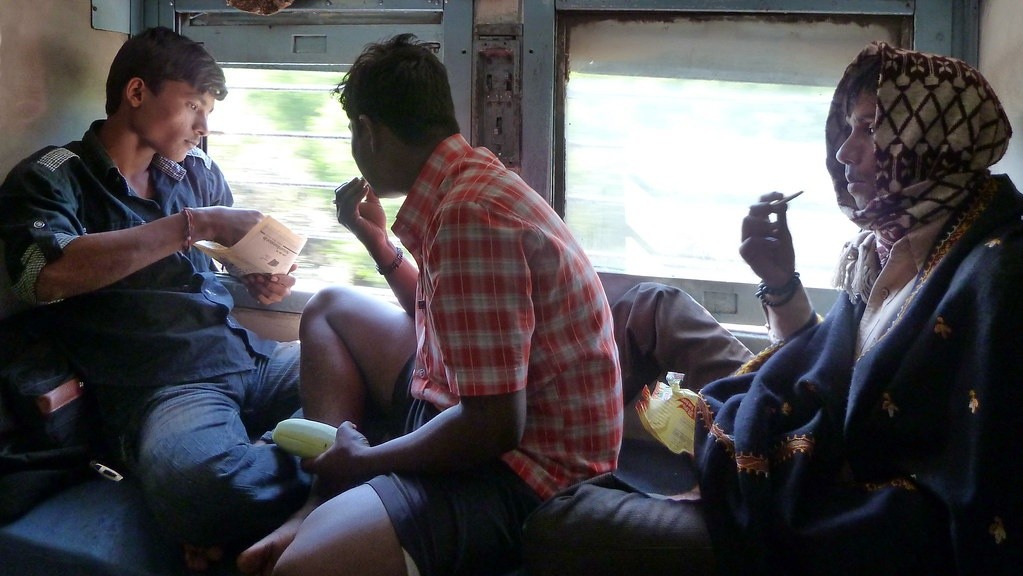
[771,191,803,206]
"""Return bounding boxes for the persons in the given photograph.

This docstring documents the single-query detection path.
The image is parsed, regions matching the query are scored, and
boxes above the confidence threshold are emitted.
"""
[243,34,623,576]
[528,40,1023,576]
[0,26,321,514]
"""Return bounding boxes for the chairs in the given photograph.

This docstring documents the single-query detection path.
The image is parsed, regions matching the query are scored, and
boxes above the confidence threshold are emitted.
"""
[0,478,189,576]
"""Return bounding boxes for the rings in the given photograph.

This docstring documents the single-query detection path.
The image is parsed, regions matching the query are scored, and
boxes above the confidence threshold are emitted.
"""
[333,198,337,204]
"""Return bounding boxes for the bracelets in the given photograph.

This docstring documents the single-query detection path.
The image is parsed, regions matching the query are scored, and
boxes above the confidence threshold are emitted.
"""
[755,272,801,305]
[181,207,194,256]
[375,247,404,275]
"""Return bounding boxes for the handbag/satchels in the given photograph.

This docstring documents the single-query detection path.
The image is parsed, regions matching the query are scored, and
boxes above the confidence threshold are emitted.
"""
[0,336,104,454]
